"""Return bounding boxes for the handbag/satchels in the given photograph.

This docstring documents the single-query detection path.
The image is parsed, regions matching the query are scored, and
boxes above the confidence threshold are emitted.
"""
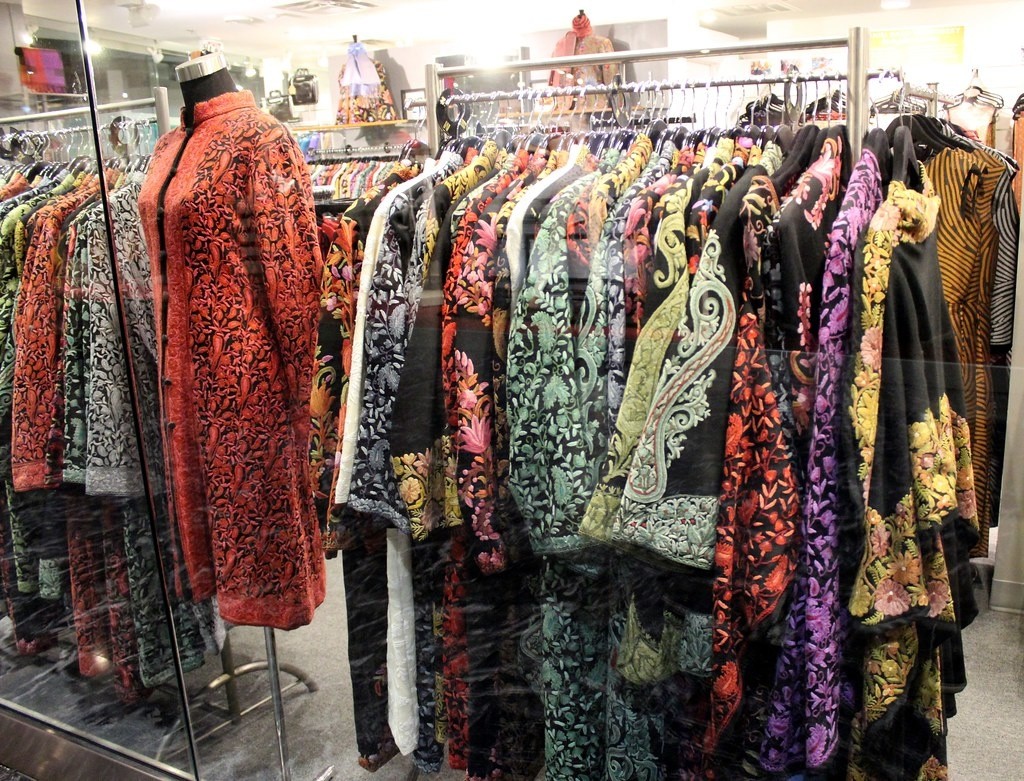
[289,67,318,104]
[265,90,293,123]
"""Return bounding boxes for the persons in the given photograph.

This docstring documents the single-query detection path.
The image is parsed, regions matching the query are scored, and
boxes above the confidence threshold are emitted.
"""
[335,42,399,123]
[549,10,624,113]
[138,52,325,630]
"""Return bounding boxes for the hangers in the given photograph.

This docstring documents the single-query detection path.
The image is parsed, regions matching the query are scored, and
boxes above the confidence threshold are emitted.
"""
[0,64,1024,190]
